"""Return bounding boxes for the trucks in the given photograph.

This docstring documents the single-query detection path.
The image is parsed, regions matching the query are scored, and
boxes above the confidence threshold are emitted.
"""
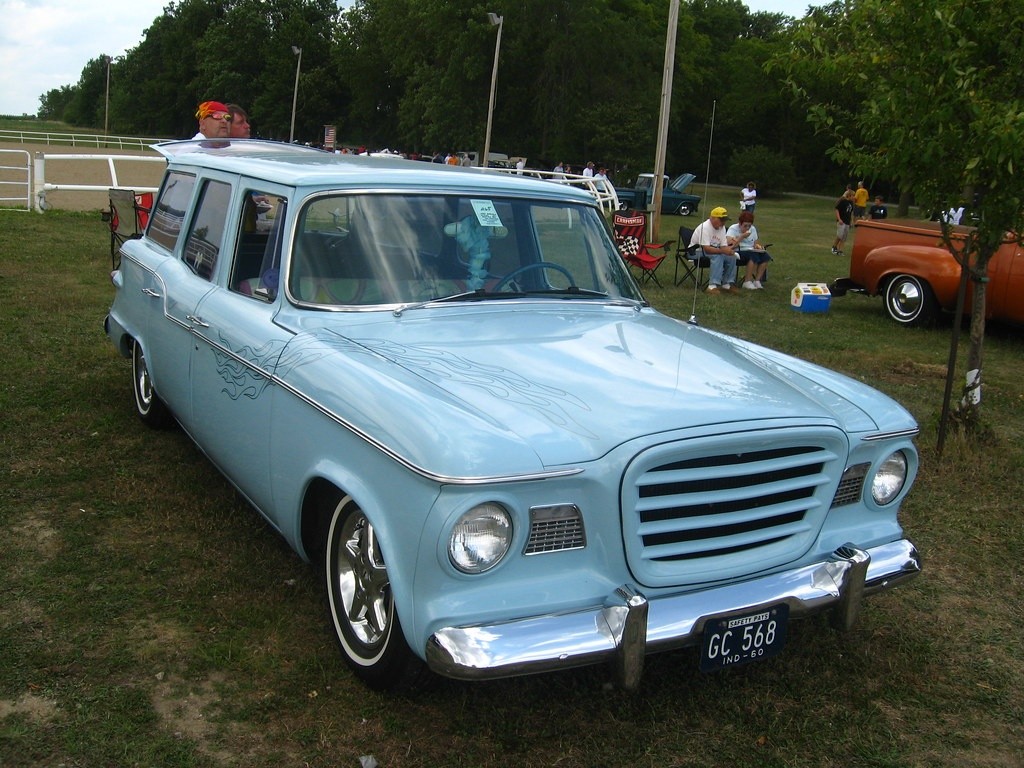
[456,151,508,168]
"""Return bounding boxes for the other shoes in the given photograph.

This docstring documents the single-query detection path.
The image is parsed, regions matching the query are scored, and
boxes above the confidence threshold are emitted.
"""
[704,287,720,296]
[837,251,845,256]
[831,246,838,255]
[719,286,739,295]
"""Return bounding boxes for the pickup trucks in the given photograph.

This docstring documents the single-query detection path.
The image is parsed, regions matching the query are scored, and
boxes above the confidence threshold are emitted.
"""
[596,173,701,217]
[829,217,1024,329]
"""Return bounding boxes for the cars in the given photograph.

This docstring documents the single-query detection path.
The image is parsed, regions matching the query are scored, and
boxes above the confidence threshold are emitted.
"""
[102,137,925,699]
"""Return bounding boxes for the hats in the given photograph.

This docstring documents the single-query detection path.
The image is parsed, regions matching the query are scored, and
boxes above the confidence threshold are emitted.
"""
[711,207,729,217]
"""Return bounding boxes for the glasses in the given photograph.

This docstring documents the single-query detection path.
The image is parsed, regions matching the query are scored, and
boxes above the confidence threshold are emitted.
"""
[742,224,751,228]
[202,112,232,121]
[719,217,728,222]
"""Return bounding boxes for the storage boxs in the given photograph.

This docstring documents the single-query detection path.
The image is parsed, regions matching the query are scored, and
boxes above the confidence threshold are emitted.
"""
[791,282,831,314]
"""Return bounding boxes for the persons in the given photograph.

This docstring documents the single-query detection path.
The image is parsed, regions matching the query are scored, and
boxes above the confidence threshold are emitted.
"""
[169,102,275,236]
[739,182,757,214]
[869,196,888,219]
[431,151,471,167]
[847,184,855,226]
[726,210,772,290]
[688,206,738,297]
[831,189,855,256]
[552,162,564,179]
[304,141,366,155]
[593,168,608,193]
[854,181,869,223]
[563,164,573,180]
[581,162,594,190]
[516,159,525,175]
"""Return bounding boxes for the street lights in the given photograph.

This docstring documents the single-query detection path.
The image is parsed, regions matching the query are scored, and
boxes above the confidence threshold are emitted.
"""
[289,46,302,143]
[104,55,113,148]
[482,11,504,169]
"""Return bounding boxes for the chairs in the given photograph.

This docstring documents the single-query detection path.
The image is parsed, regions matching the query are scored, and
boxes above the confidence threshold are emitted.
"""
[675,225,772,293]
[101,189,154,272]
[612,211,665,289]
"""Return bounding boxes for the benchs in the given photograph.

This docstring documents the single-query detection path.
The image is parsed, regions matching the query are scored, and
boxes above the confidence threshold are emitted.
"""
[236,272,518,305]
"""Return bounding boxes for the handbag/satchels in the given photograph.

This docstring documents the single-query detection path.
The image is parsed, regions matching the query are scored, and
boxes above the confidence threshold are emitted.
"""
[739,200,746,210]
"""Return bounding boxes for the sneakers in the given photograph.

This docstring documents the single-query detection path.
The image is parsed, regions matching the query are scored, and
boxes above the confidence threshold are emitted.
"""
[742,281,757,290]
[753,280,764,289]
